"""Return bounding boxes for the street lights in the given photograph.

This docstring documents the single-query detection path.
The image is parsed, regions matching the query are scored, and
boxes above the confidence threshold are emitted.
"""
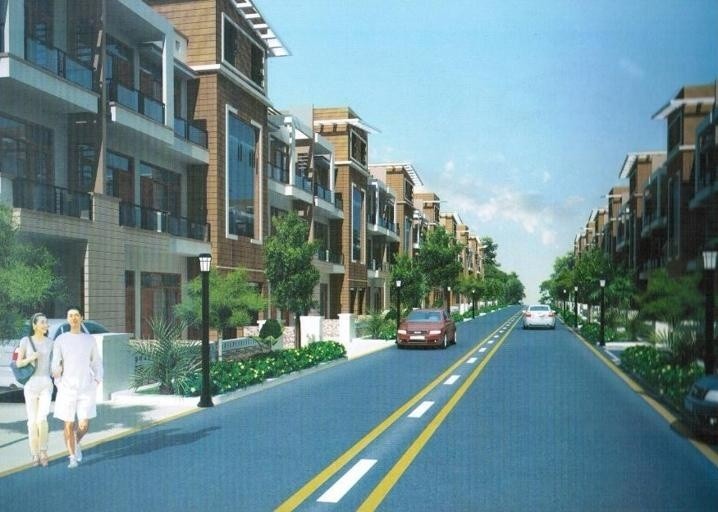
[11,358,34,385]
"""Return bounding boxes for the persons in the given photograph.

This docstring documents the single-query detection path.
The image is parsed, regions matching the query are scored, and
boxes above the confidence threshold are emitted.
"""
[16,312,63,467]
[50,306,104,469]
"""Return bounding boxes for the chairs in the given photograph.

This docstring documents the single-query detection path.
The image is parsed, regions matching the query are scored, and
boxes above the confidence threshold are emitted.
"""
[74,435,82,461]
[67,454,78,468]
